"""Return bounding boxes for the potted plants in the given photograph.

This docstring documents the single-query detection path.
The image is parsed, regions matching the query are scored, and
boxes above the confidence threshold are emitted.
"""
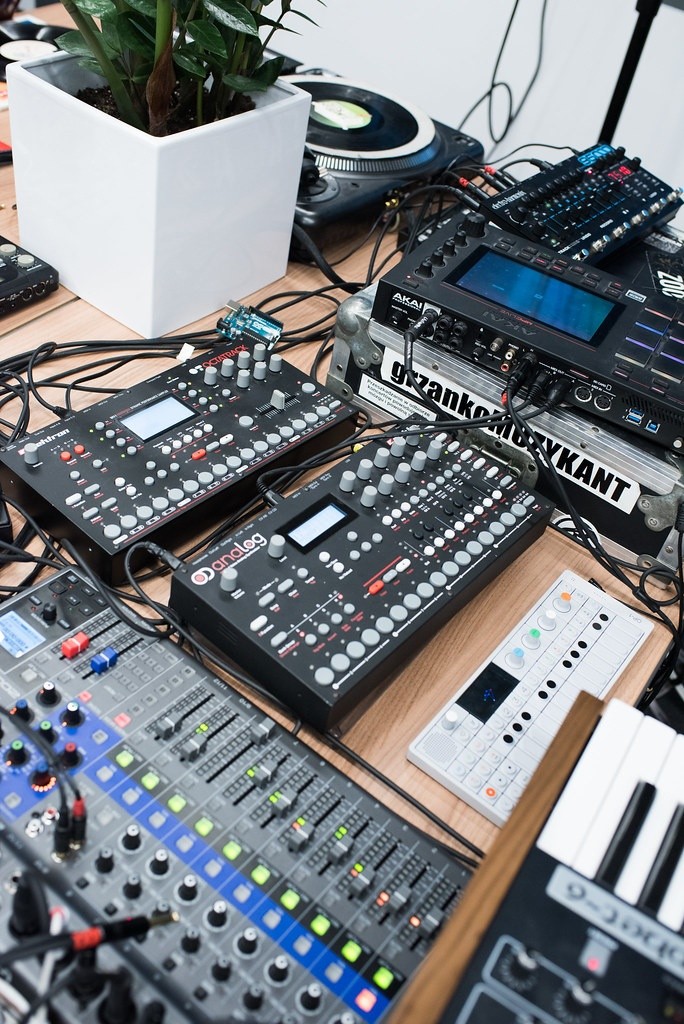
[6,0,327,344]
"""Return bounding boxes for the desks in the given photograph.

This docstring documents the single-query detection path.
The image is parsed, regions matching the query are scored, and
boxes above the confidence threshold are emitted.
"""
[0,0,682,871]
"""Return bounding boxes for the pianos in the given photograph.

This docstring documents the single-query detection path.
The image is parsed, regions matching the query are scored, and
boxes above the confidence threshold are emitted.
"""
[379,689,684,1024]
